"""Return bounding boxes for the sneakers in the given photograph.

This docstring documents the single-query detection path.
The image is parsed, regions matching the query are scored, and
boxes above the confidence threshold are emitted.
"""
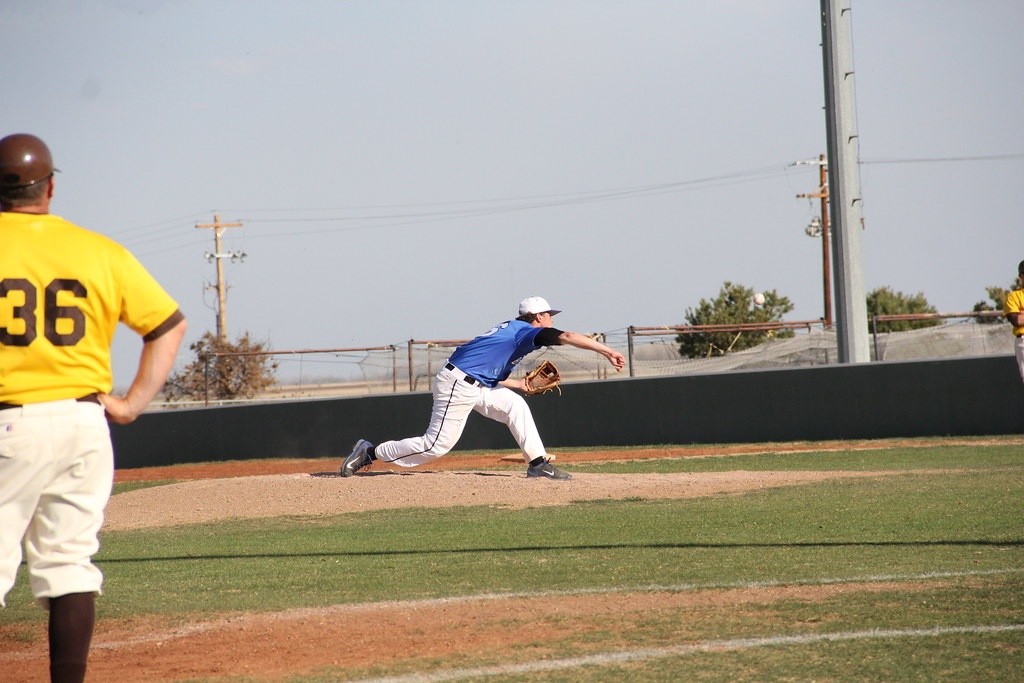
[527,457,572,480]
[341,439,373,477]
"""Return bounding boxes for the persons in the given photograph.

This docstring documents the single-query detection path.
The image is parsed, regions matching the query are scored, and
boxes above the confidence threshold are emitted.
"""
[341,297,626,481]
[0,134,188,683]
[1006,260,1024,381]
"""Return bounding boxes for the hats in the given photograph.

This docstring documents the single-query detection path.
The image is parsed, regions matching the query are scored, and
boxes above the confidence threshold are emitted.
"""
[519,296,561,316]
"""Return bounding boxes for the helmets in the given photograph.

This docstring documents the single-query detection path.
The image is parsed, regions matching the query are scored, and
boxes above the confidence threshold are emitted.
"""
[0,134,61,186]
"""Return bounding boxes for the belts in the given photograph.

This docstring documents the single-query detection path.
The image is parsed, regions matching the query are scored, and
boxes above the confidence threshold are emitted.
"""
[0,393,101,410]
[445,363,485,388]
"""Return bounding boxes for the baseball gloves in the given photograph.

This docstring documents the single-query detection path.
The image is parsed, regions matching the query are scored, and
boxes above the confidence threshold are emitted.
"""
[523,360,561,397]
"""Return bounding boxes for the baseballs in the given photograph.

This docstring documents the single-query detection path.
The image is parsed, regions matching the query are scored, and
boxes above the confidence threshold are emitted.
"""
[753,293,765,305]
